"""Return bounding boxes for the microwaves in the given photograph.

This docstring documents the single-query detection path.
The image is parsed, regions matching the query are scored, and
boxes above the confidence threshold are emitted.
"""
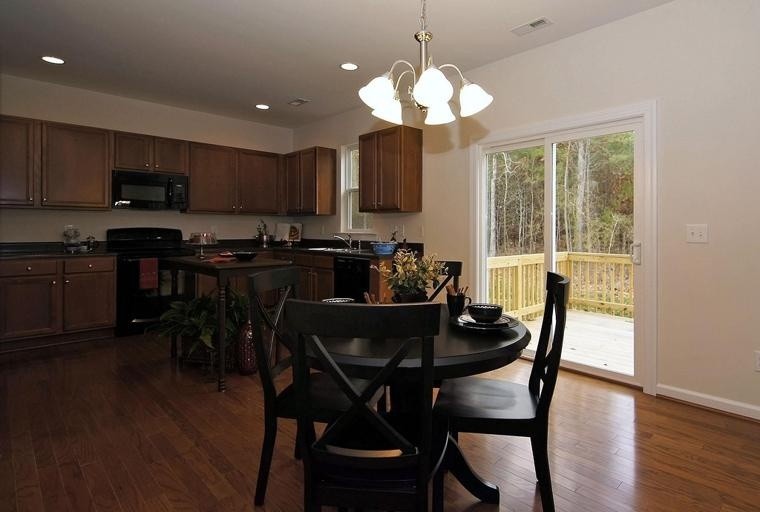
[111,170,190,213]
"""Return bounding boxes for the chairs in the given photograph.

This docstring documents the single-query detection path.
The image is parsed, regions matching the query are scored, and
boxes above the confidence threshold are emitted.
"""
[243,264,388,512]
[424,270,572,512]
[389,258,463,390]
[279,291,456,512]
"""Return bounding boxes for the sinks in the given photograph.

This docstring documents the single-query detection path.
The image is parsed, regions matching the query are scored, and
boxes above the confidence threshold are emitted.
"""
[308,248,348,252]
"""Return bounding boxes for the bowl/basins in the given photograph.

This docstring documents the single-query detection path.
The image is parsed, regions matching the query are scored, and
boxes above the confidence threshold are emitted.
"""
[370,242,398,254]
[231,252,258,260]
[466,302,504,322]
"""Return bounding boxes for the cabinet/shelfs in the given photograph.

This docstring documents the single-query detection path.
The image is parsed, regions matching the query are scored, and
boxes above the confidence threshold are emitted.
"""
[0,113,113,213]
[113,130,189,174]
[181,138,282,217]
[355,124,424,215]
[281,144,337,220]
[0,254,120,357]
[193,257,277,307]
[273,250,335,302]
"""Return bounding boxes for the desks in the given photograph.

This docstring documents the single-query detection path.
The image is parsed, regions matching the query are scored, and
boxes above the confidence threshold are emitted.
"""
[163,249,294,395]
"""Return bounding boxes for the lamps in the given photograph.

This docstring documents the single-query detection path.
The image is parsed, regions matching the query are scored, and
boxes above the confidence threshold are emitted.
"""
[358,0,495,128]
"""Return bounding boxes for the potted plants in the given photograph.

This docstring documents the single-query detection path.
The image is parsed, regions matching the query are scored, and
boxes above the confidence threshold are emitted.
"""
[159,287,245,377]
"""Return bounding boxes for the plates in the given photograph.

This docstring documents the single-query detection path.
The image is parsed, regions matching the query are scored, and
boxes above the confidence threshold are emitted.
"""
[452,313,520,332]
[218,252,233,257]
[321,297,355,303]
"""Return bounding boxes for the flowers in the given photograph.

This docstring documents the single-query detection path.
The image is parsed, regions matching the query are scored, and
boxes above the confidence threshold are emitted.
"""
[369,246,449,292]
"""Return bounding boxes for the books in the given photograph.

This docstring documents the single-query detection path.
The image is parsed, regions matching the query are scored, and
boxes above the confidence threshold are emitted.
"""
[272,222,303,243]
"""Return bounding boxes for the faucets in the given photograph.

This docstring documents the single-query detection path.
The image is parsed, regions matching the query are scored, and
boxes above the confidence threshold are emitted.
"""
[333,234,352,248]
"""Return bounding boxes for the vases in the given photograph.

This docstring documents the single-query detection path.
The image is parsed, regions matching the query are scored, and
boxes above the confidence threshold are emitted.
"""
[387,288,429,304]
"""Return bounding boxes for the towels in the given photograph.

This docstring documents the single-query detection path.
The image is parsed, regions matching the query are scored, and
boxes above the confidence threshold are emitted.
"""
[138,257,159,290]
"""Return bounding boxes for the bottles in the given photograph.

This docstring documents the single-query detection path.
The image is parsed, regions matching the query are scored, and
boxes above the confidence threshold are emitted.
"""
[234,303,259,376]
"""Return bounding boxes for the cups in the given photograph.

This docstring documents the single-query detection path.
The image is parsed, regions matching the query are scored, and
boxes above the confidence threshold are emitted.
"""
[258,235,270,249]
[445,293,471,314]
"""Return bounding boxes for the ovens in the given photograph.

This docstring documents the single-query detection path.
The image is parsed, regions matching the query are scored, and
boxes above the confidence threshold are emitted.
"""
[117,256,187,336]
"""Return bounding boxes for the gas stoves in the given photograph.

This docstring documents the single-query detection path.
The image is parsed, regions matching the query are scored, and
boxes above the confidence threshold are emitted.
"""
[105,248,190,256]
